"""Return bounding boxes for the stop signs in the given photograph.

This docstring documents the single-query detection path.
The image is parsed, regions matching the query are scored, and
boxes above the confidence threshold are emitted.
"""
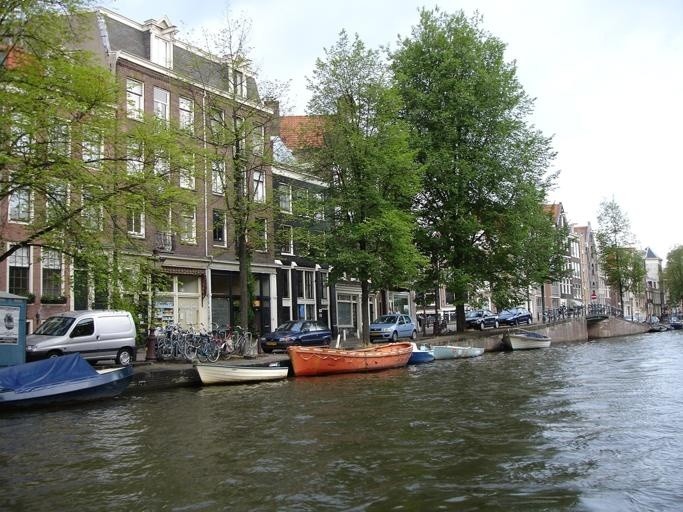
[591,295,596,299]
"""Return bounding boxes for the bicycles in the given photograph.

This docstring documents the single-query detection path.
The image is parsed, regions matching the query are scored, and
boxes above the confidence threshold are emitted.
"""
[156,322,249,363]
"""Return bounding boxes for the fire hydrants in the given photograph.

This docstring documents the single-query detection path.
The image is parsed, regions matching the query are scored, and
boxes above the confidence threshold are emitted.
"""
[144,326,156,360]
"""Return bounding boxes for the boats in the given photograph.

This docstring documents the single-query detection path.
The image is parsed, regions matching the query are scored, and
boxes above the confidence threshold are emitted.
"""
[431,344,484,360]
[191,360,288,384]
[408,342,435,363]
[0,350,132,410]
[648,322,683,332]
[502,327,551,350]
[287,341,413,377]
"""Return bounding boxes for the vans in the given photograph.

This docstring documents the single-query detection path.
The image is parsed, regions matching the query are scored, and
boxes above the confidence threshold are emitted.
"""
[25,309,136,365]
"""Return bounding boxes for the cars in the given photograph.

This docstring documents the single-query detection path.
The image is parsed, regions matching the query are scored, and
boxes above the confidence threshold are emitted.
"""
[260,320,332,353]
[624,315,638,321]
[464,310,500,331]
[369,314,417,342]
[498,306,532,326]
[647,316,660,323]
[670,316,680,322]
